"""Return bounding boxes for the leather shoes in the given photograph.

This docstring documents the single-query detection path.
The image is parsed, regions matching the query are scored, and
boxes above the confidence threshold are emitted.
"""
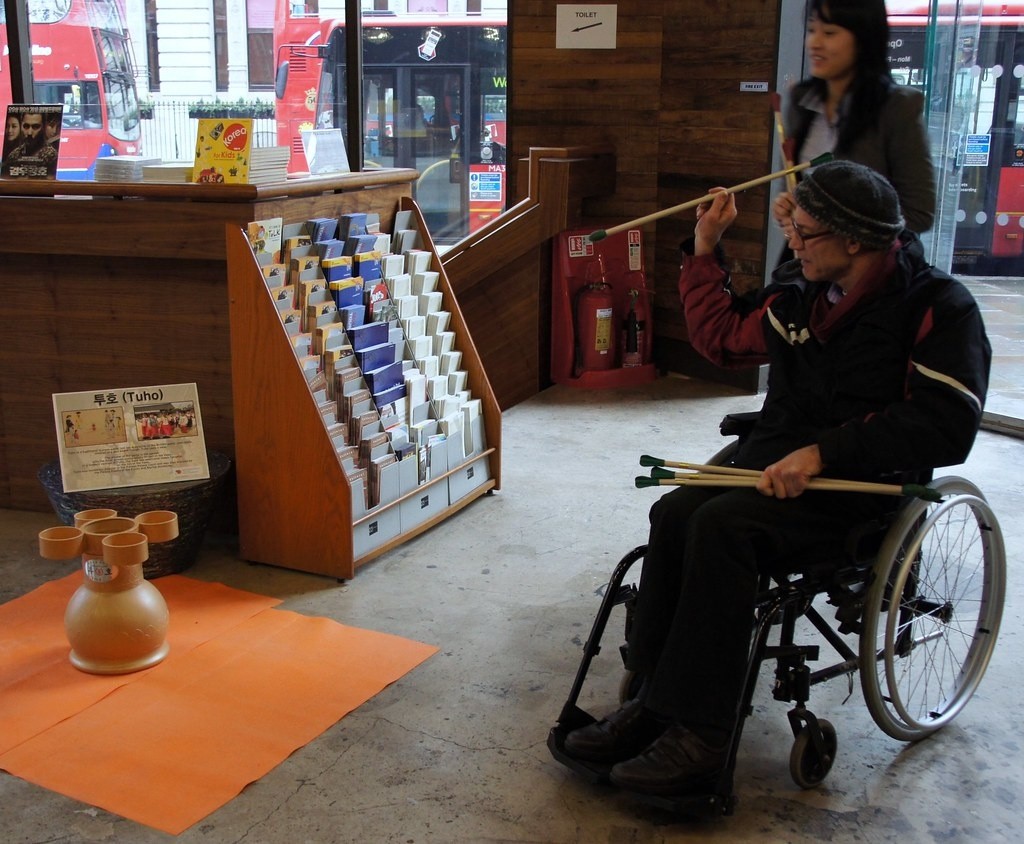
[564,698,672,761]
[609,723,730,793]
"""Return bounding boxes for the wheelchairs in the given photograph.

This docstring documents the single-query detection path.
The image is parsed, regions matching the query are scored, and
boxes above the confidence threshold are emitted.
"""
[547,402,1008,818]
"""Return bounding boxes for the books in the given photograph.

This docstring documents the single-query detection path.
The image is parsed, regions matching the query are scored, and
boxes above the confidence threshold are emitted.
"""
[93,118,291,184]
[247,210,482,508]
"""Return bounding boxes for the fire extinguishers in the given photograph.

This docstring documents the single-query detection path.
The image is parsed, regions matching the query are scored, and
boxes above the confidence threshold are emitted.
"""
[573,270,616,371]
[621,277,656,368]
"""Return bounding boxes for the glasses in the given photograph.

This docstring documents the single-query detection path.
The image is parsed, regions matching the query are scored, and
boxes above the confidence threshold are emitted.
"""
[791,217,837,247]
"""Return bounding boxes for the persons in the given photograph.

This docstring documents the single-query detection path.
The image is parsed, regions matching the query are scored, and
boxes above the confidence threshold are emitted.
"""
[4,112,61,167]
[567,159,993,792]
[789,0,937,235]
[388,105,431,156]
[137,411,193,437]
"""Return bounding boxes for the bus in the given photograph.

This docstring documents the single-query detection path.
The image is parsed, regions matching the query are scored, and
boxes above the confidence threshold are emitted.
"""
[0,0,143,198]
[273,0,1024,277]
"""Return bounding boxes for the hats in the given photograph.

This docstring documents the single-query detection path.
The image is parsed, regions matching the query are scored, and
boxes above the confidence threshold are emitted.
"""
[792,161,905,249]
